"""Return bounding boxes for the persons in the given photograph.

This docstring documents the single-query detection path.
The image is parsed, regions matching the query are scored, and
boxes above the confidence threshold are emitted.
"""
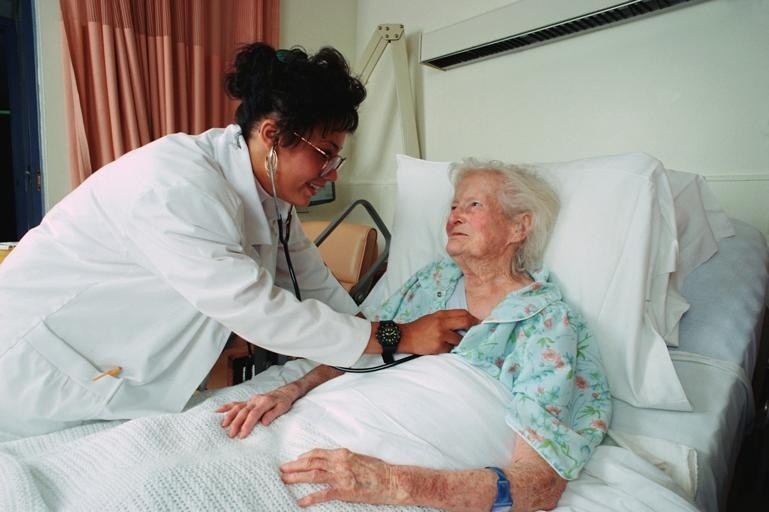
[215,154,615,512]
[0,39,487,440]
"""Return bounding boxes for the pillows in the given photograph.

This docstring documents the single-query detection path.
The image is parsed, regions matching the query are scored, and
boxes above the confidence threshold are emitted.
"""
[652,167,735,348]
[392,155,693,413]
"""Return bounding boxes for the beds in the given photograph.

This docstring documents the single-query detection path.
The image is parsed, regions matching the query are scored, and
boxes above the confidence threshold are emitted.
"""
[1,214,768,512]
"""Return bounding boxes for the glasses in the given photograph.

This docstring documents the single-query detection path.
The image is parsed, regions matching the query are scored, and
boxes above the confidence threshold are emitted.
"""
[290,132,346,177]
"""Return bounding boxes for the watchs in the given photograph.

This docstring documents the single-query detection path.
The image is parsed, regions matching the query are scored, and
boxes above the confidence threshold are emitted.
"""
[375,321,401,364]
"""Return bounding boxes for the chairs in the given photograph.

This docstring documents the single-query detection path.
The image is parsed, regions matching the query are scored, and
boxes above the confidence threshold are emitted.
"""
[207,219,379,390]
